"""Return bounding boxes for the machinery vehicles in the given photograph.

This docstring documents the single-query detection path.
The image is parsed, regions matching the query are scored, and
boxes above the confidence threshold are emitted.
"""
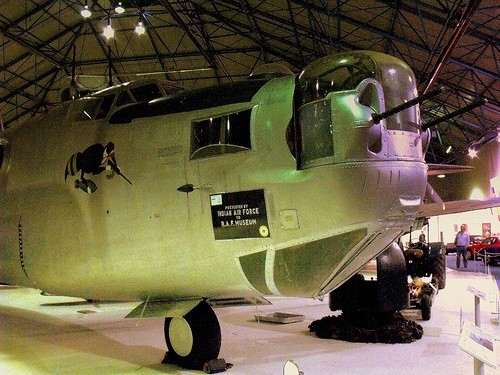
[398,217,448,320]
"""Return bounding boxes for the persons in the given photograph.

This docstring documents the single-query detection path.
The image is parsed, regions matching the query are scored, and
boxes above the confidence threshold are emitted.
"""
[485,228,491,236]
[454,225,470,268]
[419,231,426,243]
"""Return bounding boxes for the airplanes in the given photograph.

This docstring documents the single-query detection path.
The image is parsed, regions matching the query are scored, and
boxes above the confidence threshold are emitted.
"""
[0,49,500,373]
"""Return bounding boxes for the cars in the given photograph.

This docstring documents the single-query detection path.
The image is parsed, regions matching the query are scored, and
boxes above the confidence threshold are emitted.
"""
[467,237,500,260]
[477,247,500,265]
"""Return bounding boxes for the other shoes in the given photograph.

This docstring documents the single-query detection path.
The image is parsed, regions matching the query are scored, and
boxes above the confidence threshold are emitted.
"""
[457,266,460,268]
[464,266,468,268]
